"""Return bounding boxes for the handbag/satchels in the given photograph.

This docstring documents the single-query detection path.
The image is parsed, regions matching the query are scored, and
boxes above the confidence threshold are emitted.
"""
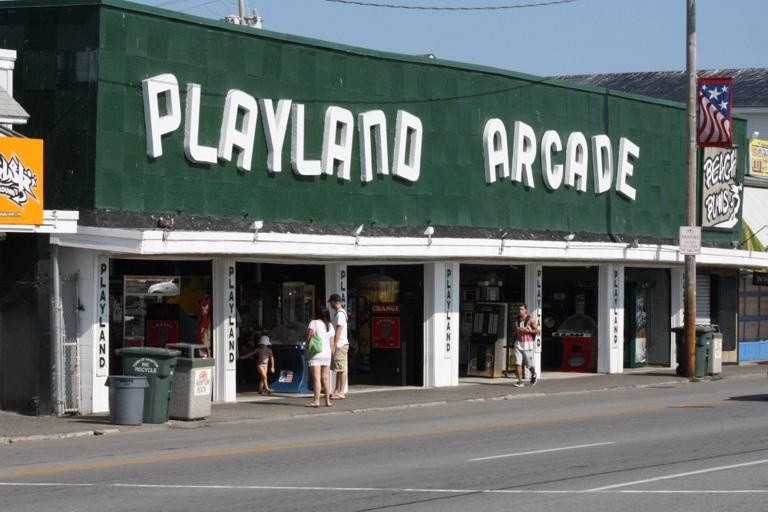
[303,320,322,361]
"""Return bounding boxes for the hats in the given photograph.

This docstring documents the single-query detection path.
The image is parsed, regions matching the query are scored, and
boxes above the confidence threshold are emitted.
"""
[328,294,340,302]
[257,336,272,346]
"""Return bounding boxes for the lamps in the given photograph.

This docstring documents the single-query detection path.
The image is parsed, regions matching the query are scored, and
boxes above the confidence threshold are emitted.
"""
[249,220,263,242]
[563,234,575,248]
[626,239,638,249]
[424,227,434,245]
[352,224,363,245]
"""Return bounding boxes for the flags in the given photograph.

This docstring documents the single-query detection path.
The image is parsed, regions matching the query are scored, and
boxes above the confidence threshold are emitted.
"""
[694,77,735,148]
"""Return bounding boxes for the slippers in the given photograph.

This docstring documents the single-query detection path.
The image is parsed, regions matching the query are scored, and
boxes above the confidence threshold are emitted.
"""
[304,393,345,407]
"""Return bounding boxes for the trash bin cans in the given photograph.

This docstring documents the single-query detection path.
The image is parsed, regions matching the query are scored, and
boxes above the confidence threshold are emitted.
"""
[104,343,214,425]
[670,324,724,377]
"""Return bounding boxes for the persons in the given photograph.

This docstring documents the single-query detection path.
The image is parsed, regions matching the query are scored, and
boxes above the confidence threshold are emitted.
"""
[324,294,352,400]
[237,335,276,395]
[302,307,334,407]
[236,305,242,362]
[511,303,540,387]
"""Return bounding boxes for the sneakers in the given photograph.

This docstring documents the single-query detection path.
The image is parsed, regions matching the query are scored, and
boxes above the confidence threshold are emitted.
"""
[513,381,524,387]
[531,373,537,385]
